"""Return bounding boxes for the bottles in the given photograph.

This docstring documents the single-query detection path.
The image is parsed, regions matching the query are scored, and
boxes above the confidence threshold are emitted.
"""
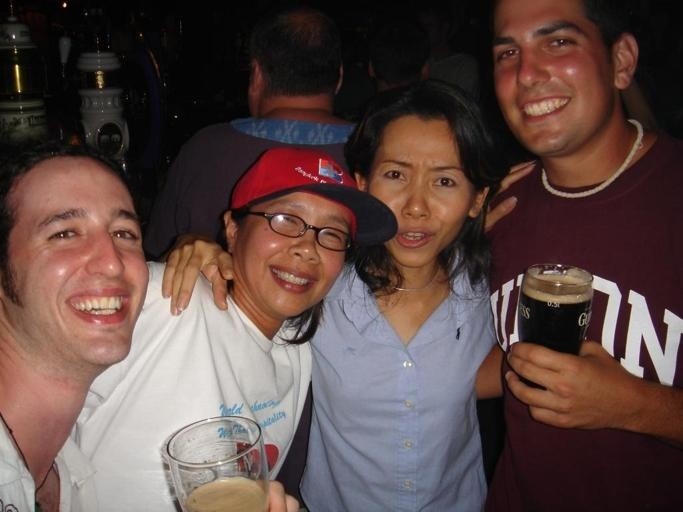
[0,0,128,175]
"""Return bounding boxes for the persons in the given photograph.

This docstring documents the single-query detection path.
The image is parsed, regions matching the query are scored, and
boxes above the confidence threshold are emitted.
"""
[620,71,661,138]
[1,140,302,511]
[342,15,431,121]
[160,76,536,511]
[474,0,683,512]
[139,5,363,510]
[52,145,538,510]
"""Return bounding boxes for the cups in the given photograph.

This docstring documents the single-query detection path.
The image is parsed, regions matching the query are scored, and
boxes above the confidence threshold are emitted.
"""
[162,415,271,510]
[519,264,596,358]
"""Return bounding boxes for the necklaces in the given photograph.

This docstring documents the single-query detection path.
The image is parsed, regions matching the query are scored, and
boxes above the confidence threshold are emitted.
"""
[390,262,442,293]
[1,413,55,511]
[541,117,645,198]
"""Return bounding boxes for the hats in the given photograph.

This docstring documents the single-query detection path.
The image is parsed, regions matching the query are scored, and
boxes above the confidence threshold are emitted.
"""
[228,142,400,251]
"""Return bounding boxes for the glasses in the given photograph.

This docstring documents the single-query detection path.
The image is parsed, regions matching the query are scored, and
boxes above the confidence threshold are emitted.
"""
[268,210,354,254]
